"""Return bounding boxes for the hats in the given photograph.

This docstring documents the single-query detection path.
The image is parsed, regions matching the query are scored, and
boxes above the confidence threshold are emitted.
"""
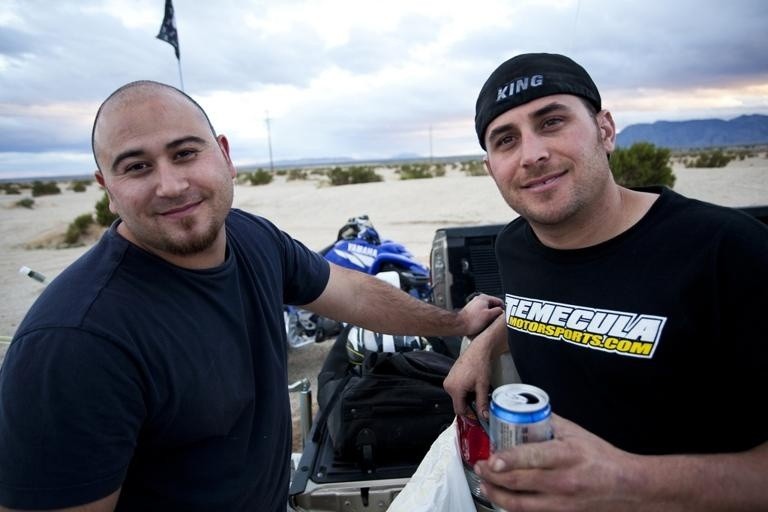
[474,52,603,151]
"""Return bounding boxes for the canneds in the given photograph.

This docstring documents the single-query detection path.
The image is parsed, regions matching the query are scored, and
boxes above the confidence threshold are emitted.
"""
[489,383,553,453]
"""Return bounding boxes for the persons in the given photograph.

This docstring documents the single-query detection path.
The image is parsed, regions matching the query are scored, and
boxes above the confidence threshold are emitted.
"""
[441,54,767,510]
[1,80,506,510]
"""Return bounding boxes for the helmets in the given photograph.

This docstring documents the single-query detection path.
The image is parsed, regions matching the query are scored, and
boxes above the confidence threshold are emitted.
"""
[346,325,432,378]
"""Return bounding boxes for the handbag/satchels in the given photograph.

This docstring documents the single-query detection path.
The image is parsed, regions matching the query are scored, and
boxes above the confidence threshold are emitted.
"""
[316,318,457,466]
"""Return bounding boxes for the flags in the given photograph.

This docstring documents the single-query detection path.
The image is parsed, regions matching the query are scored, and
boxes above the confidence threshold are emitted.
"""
[154,1,180,60]
